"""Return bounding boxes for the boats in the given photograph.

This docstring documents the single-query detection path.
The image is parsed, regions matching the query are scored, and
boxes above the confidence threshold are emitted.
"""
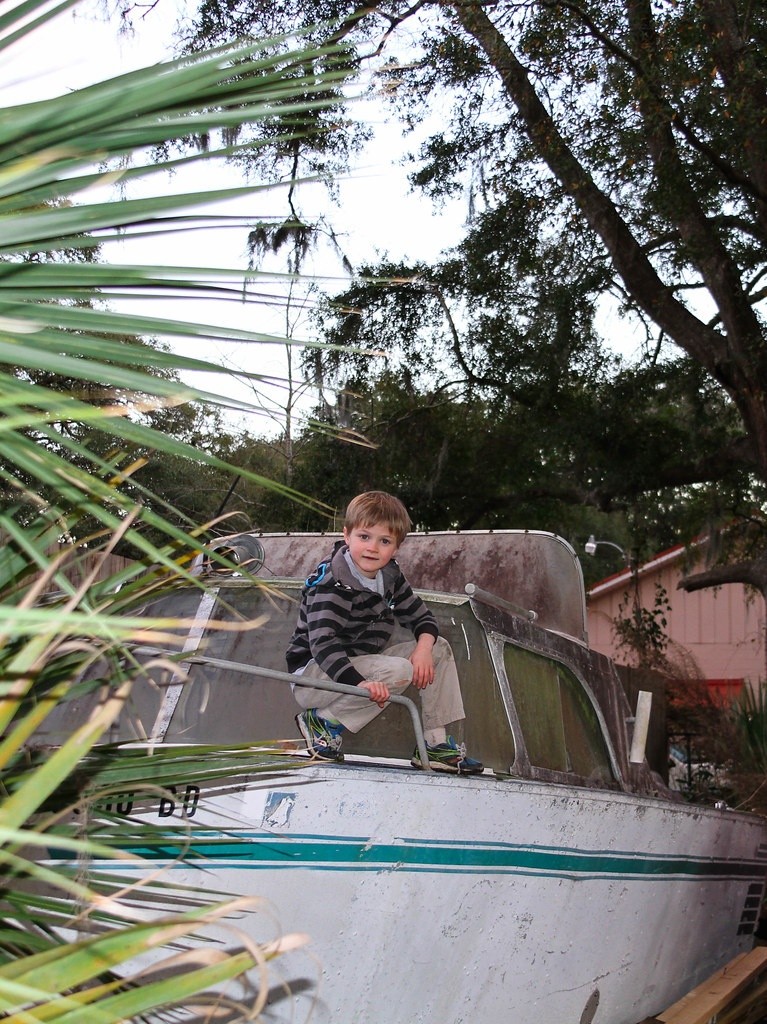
[0,529,767,1023]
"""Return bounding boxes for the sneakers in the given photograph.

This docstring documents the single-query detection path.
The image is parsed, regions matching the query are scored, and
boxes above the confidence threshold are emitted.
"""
[411,735,484,775]
[294,709,344,762]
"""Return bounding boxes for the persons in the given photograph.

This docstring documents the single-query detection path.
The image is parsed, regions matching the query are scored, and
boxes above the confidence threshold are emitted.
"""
[286,492,484,775]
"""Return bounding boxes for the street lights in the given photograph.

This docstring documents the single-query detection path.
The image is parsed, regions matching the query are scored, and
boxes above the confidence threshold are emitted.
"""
[585,535,633,570]
